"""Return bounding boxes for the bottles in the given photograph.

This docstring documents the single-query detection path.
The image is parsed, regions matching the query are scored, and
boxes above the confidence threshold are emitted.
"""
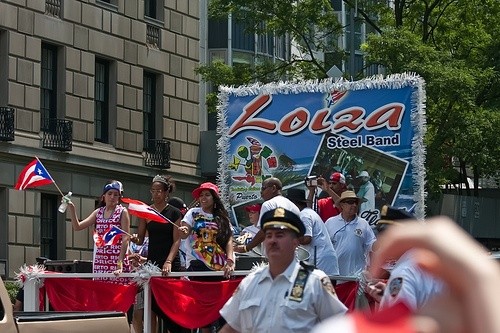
[58,192,72,213]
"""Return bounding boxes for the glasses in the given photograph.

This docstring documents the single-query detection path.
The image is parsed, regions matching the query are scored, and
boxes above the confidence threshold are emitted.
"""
[261,185,272,191]
[150,189,167,195]
[341,200,360,205]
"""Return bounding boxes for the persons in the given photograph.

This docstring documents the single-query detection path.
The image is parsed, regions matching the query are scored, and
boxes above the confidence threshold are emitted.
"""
[346,170,383,214]
[16,286,24,310]
[68,173,500,333]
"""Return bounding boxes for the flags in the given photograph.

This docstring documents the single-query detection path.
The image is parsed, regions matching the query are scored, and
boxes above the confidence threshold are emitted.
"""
[92,227,125,248]
[14,159,53,190]
[121,197,168,223]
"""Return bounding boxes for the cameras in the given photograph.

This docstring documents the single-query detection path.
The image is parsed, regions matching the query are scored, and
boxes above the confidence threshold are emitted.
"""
[305,174,322,187]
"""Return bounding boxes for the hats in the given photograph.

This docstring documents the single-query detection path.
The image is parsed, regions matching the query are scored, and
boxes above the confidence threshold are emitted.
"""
[246,204,262,213]
[334,190,368,209]
[192,182,219,201]
[356,171,370,178]
[260,206,306,238]
[329,173,348,190]
[375,204,419,224]
